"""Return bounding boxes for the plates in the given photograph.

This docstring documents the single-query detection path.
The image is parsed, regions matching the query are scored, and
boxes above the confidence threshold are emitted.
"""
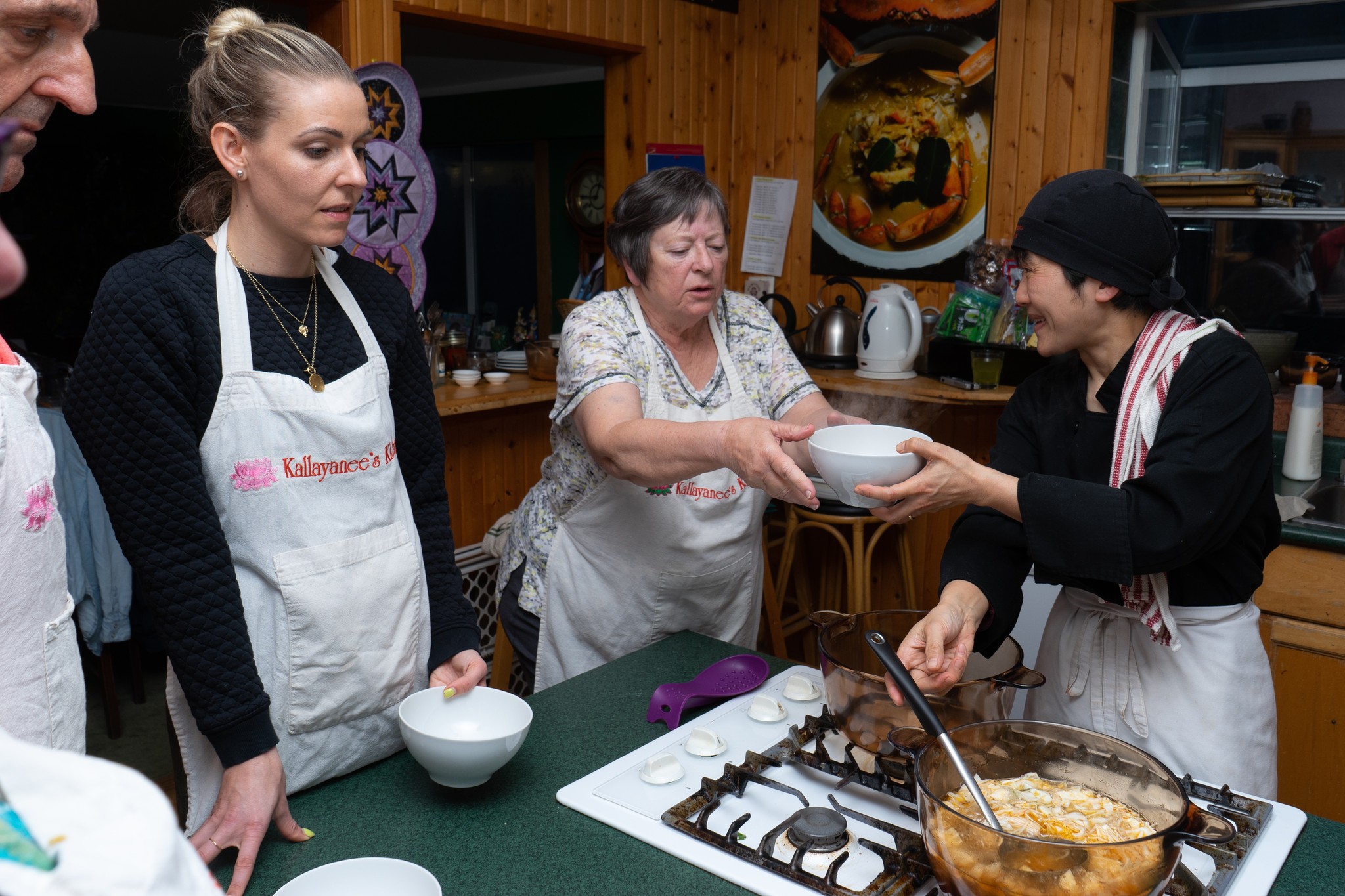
[272,856,441,896]
[494,351,528,372]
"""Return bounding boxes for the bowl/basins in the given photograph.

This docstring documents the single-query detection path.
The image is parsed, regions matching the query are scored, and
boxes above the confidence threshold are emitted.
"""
[483,373,510,385]
[811,32,993,270]
[809,424,932,508]
[452,370,481,387]
[1280,352,1341,387]
[1240,329,1297,374]
[397,686,533,789]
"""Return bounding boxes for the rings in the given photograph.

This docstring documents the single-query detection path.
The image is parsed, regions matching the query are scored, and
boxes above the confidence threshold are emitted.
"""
[209,838,222,851]
[909,515,915,520]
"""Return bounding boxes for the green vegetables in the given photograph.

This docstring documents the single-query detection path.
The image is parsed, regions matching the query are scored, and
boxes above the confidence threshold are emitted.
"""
[848,124,952,210]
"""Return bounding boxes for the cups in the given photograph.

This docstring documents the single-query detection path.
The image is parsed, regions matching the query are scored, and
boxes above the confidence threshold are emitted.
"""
[425,345,439,385]
[467,358,486,369]
[971,348,1004,390]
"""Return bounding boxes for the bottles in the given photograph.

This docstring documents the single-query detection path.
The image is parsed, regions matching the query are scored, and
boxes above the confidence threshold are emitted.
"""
[914,306,942,374]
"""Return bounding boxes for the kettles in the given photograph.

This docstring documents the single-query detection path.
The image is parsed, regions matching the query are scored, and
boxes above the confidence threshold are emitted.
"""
[803,275,867,369]
[759,293,805,363]
[854,284,922,380]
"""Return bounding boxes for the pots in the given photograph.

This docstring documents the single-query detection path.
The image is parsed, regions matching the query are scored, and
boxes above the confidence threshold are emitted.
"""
[809,609,1047,761]
[888,718,1236,896]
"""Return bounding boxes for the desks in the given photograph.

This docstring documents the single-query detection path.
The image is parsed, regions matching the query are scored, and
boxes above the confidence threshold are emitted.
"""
[810,360,1019,407]
[434,370,556,417]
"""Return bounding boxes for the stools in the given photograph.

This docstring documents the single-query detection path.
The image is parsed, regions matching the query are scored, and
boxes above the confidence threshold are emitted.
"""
[757,498,918,683]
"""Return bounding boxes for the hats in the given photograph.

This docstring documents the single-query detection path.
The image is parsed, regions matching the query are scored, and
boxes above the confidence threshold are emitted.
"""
[1011,168,1185,304]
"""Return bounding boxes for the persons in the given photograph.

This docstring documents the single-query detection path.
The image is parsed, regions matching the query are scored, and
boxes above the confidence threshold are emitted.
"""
[496,169,873,695]
[0,0,98,757]
[856,170,1277,805]
[60,8,486,896]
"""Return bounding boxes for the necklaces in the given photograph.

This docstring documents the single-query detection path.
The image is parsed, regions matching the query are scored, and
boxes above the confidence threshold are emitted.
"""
[226,244,315,338]
[228,246,326,393]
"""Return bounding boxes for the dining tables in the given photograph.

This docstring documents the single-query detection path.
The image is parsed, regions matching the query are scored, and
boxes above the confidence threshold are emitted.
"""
[210,632,1345,896]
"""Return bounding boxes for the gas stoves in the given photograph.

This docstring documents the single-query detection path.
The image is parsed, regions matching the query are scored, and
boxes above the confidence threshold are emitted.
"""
[556,665,1307,896]
